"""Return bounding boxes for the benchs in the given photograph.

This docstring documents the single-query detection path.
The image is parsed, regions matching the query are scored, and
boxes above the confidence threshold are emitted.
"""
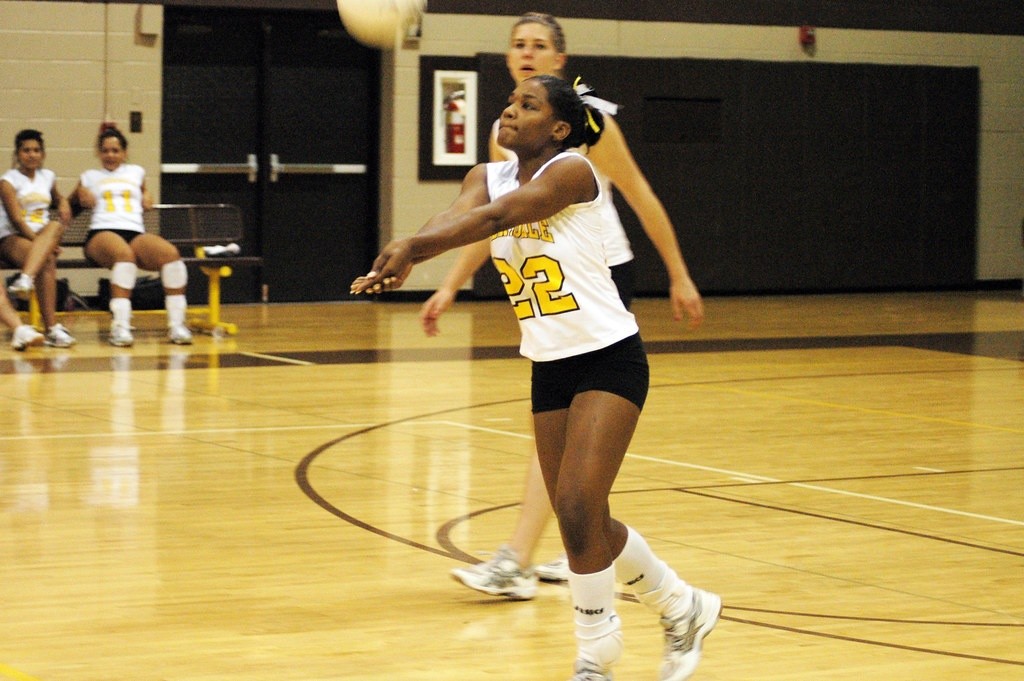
[0,202,265,336]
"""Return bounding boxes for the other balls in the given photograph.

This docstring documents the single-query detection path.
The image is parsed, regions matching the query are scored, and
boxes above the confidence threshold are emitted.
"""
[337,0,427,46]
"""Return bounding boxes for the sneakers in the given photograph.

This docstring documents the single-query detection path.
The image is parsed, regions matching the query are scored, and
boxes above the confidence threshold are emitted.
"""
[167,325,194,345]
[7,277,38,303]
[534,556,572,582]
[108,322,136,347]
[11,326,46,352]
[40,325,78,349]
[450,548,538,601]
[655,584,722,680]
[570,650,617,681]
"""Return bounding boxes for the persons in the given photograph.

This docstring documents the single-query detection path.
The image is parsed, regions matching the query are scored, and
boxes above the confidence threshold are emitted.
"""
[0,285,44,350]
[421,11,704,600]
[0,130,75,347]
[349,74,722,681]
[67,121,193,346]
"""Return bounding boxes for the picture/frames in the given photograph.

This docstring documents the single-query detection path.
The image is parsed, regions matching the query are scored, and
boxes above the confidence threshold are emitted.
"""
[419,56,492,179]
